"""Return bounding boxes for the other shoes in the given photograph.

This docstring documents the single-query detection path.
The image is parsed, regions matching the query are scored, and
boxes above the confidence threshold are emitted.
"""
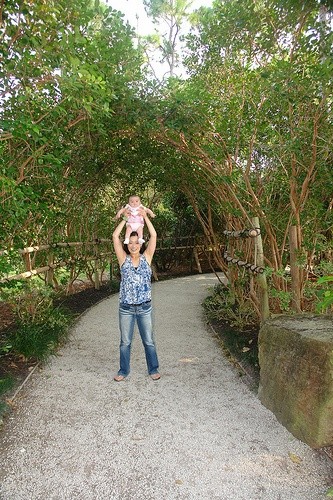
[114,374,124,381]
[151,373,161,380]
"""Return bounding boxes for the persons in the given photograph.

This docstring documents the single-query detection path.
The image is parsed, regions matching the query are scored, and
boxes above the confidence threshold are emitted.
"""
[114,194,156,245]
[113,209,162,382]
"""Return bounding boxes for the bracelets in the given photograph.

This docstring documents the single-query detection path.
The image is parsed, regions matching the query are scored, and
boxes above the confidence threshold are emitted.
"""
[123,216,128,222]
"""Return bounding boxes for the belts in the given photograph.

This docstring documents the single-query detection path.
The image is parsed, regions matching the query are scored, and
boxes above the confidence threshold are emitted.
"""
[125,300,151,306]
